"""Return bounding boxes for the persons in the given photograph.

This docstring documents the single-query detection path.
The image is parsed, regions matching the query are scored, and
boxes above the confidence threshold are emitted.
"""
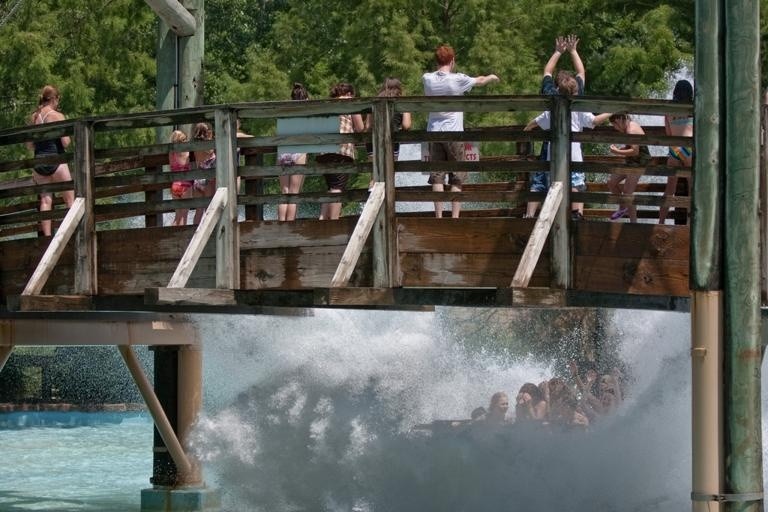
[26,86,75,237]
[523,34,694,225]
[441,359,624,438]
[420,45,500,218]
[168,77,412,227]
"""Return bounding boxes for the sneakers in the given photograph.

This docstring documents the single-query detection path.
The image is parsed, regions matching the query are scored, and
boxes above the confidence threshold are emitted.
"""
[609,207,629,220]
